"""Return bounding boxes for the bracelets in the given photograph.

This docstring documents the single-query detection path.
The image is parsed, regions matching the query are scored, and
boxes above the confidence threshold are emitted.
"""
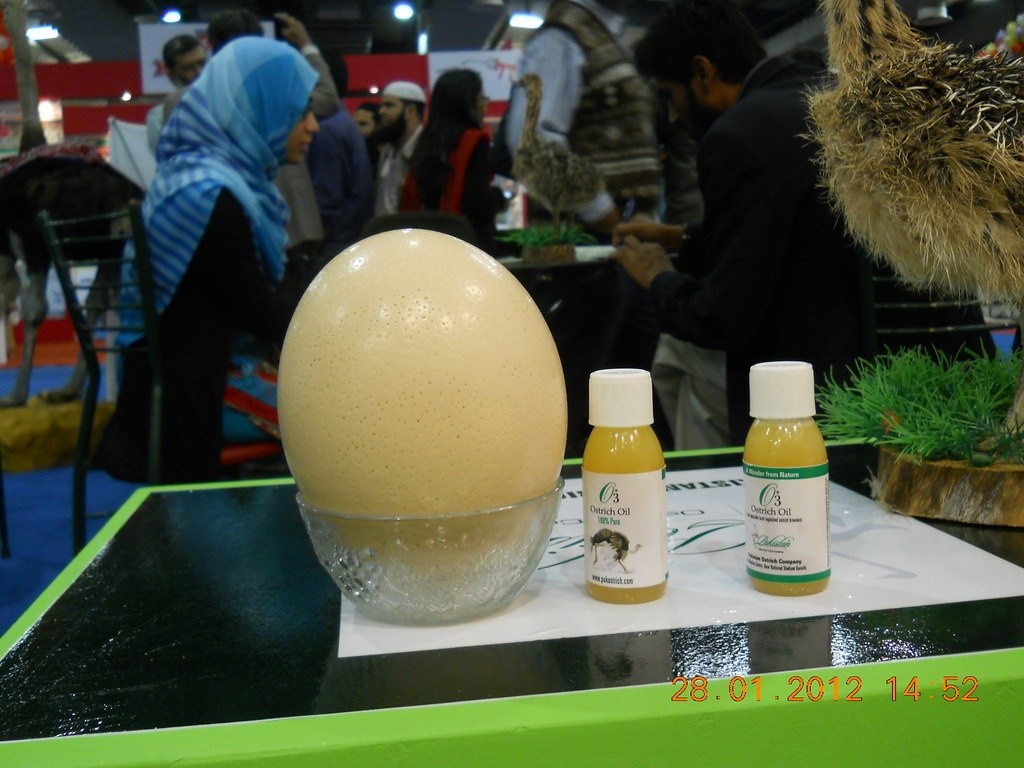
[679,221,690,247]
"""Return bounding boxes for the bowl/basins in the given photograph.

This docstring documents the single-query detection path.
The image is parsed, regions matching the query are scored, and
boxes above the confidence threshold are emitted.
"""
[291,474,564,623]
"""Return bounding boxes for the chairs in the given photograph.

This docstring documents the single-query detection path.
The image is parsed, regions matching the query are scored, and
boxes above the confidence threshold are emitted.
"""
[38,204,181,533]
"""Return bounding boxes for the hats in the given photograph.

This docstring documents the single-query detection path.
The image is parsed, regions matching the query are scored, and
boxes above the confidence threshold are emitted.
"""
[381,81,426,105]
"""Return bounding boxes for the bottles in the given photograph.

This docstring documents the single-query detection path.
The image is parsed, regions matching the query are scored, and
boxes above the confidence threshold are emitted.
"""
[746,359,832,597]
[585,369,669,605]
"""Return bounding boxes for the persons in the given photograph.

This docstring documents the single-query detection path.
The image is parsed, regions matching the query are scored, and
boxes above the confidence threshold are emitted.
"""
[91,36,339,485]
[396,69,509,249]
[377,81,428,213]
[505,1,664,243]
[164,10,336,330]
[144,36,211,150]
[352,104,379,161]
[312,53,377,245]
[608,0,884,448]
